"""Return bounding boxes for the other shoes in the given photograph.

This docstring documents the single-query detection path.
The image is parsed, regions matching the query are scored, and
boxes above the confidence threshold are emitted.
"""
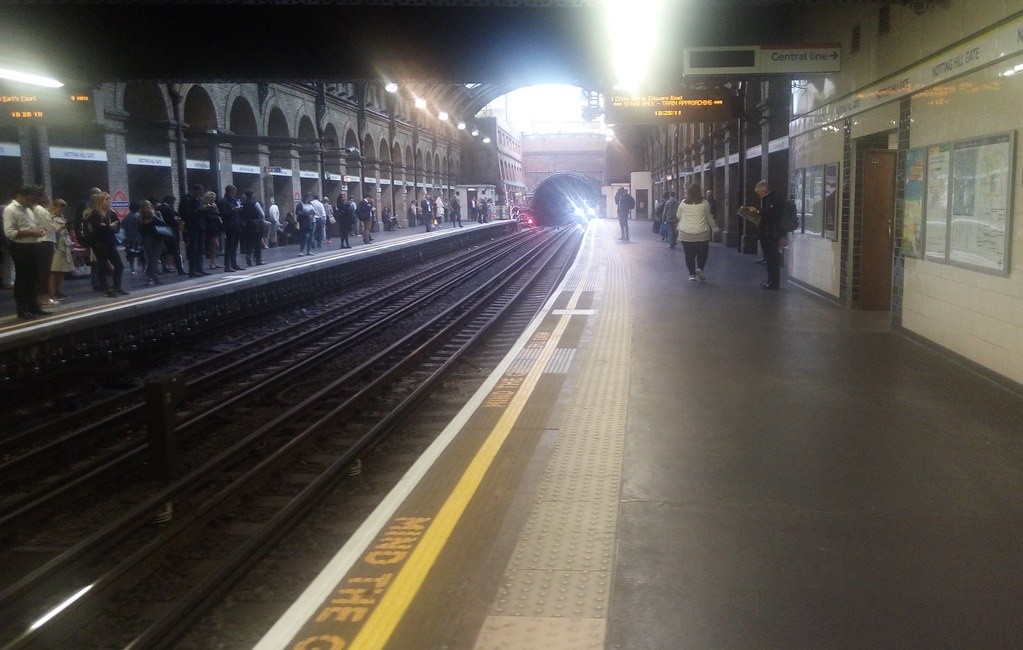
[664,238,668,241]
[661,236,665,240]
[622,238,629,241]
[669,244,674,249]
[673,241,677,246]
[760,281,769,286]
[617,237,625,240]
[761,284,777,289]
[3,234,375,319]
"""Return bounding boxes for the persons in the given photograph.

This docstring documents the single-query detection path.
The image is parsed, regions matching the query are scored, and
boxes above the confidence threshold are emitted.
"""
[750,180,784,291]
[675,184,720,282]
[0,181,494,321]
[615,187,716,249]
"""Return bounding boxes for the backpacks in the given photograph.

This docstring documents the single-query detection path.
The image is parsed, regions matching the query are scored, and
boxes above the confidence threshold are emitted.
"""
[776,198,798,232]
[354,204,366,221]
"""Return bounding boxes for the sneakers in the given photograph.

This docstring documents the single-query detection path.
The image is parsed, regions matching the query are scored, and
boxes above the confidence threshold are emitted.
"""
[695,268,705,281]
[688,274,696,281]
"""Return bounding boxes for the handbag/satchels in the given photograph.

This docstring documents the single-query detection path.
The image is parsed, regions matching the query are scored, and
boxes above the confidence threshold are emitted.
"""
[152,210,175,241]
[659,223,667,237]
[651,219,659,235]
